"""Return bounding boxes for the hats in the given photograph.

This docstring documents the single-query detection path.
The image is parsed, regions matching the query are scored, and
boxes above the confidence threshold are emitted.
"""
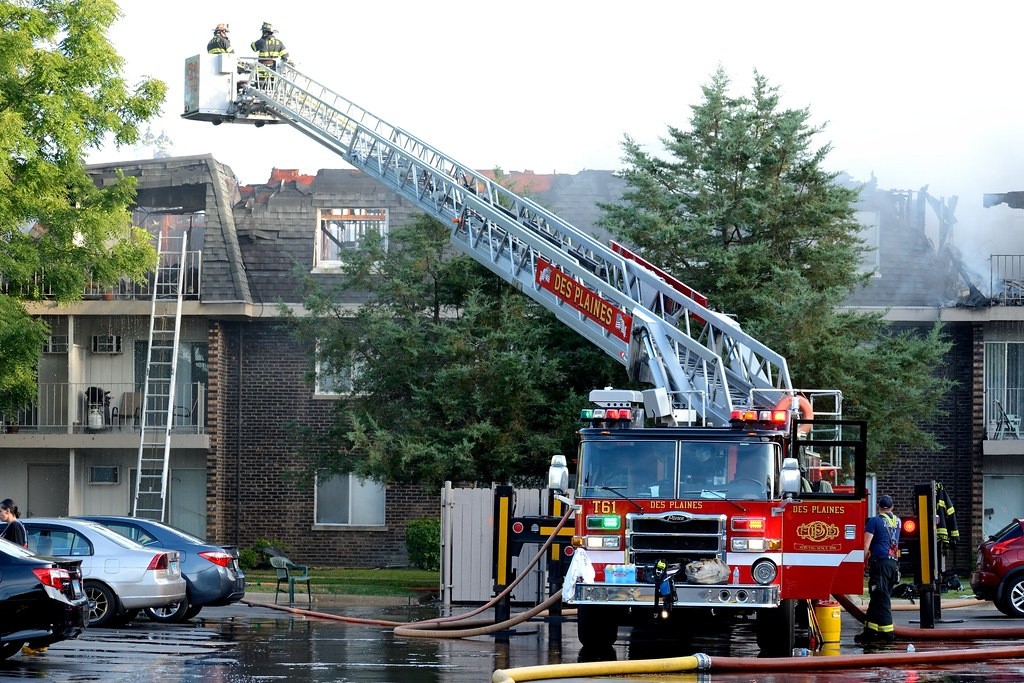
[879,495,892,509]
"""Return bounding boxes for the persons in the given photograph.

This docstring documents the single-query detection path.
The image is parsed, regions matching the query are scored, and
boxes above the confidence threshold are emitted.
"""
[854,495,901,644]
[0,499,49,654]
[250,22,295,91]
[208,24,233,54]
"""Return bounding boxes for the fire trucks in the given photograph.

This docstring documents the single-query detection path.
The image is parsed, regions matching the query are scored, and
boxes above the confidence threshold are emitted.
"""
[179,48,875,657]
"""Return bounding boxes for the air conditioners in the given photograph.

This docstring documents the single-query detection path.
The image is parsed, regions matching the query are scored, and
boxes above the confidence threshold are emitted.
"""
[92,334,121,354]
[41,335,68,353]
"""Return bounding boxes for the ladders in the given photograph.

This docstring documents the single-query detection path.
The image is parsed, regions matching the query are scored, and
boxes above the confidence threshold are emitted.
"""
[132,230,188,521]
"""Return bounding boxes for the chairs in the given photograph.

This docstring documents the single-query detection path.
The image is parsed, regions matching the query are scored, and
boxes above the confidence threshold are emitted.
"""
[994,400,1022,440]
[110,391,146,429]
[270,555,312,609]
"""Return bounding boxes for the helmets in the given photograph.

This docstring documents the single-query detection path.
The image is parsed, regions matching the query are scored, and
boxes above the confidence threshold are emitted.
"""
[216,24,229,32]
[262,23,273,35]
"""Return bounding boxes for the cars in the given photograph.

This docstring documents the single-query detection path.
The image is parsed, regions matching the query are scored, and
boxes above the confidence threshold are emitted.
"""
[0,519,187,628]
[69,514,246,624]
[0,550,92,660]
[968,517,1024,618]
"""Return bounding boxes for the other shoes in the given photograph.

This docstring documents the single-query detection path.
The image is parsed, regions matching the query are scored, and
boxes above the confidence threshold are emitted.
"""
[35,647,47,652]
[22,646,37,653]
[854,630,896,642]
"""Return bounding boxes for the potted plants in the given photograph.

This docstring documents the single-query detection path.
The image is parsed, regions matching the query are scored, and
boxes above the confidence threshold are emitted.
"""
[103,283,113,300]
